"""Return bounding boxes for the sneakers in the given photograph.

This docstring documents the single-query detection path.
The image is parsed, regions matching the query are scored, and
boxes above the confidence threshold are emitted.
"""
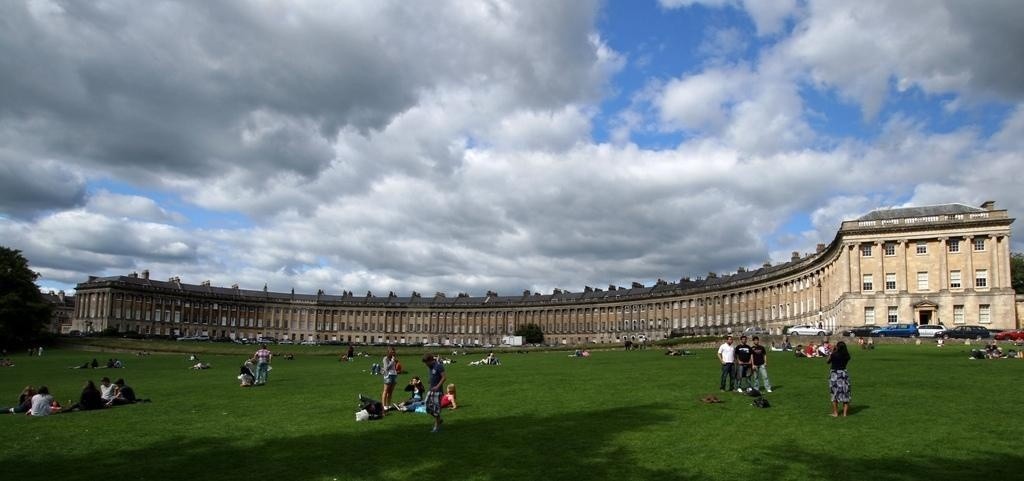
[432,418,443,427]
[430,426,440,432]
[722,386,772,392]
[384,402,405,413]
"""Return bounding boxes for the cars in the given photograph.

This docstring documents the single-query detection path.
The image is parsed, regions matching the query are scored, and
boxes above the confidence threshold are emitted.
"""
[935,326,989,342]
[742,326,769,336]
[786,325,833,337]
[71,331,81,337]
[994,328,1024,343]
[86,331,102,336]
[843,326,881,339]
[871,323,920,338]
[120,331,147,339]
[909,325,947,340]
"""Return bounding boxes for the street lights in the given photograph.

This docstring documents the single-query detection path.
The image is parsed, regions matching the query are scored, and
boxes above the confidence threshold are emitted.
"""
[816,278,822,329]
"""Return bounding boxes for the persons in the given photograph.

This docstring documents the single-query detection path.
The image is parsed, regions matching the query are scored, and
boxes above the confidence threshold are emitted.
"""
[717,336,737,393]
[734,336,752,393]
[826,342,851,418]
[966,337,1024,360]
[339,345,354,363]
[857,335,874,352]
[624,341,633,351]
[576,348,589,358]
[665,344,691,356]
[357,345,458,433]
[237,343,272,387]
[751,336,773,392]
[768,335,833,359]
[188,353,211,370]
[1,376,135,417]
[27,345,43,358]
[467,351,502,367]
[283,351,294,361]
[0,348,16,368]
[936,336,945,352]
[135,349,152,357]
[70,356,122,370]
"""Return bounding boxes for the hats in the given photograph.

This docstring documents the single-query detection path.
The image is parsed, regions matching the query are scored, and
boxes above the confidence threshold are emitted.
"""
[703,395,721,402]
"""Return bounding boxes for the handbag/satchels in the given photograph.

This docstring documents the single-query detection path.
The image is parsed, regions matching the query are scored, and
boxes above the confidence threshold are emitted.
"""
[752,396,770,407]
[744,386,761,396]
